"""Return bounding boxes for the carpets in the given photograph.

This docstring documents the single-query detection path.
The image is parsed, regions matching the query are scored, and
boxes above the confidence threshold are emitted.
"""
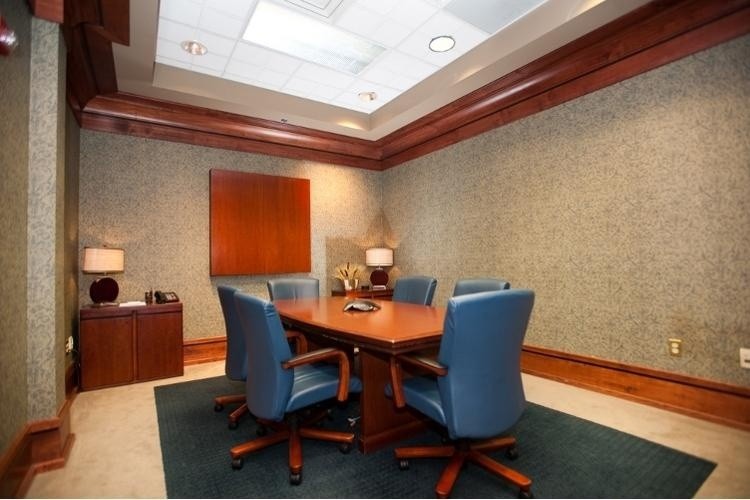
[154,373,720,499]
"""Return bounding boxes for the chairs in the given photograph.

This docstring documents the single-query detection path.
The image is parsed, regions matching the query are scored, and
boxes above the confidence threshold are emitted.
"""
[452,278,509,298]
[390,276,438,307]
[383,288,539,498]
[268,278,320,303]
[214,285,312,429]
[231,292,364,486]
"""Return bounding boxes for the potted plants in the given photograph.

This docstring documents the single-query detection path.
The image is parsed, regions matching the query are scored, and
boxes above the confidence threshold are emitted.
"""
[331,262,361,290]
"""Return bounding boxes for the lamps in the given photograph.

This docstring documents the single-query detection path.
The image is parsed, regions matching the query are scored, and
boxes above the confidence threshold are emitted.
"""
[365,247,394,290]
[80,244,126,302]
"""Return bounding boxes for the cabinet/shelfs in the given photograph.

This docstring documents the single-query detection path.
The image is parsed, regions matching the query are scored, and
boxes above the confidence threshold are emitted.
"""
[332,287,392,301]
[78,300,184,392]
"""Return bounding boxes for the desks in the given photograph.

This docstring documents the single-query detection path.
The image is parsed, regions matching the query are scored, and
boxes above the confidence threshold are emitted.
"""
[270,295,450,454]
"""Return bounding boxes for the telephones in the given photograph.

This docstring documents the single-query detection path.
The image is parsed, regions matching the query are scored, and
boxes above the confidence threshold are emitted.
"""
[343,300,381,311]
[154,290,179,304]
